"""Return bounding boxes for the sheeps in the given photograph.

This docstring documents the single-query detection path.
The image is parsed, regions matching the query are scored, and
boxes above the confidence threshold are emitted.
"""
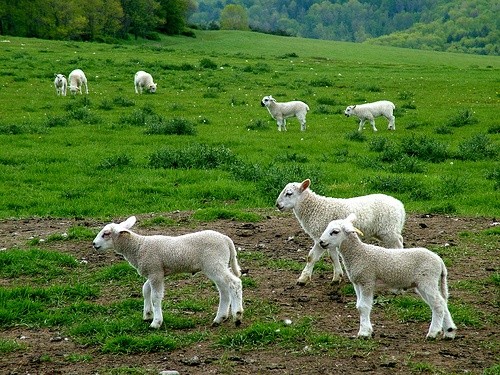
[344,100,395,132]
[54,73,68,97]
[275,178,406,295]
[93,215,249,331]
[66,68,89,94]
[261,95,310,132]
[318,213,457,342]
[134,70,157,94]
